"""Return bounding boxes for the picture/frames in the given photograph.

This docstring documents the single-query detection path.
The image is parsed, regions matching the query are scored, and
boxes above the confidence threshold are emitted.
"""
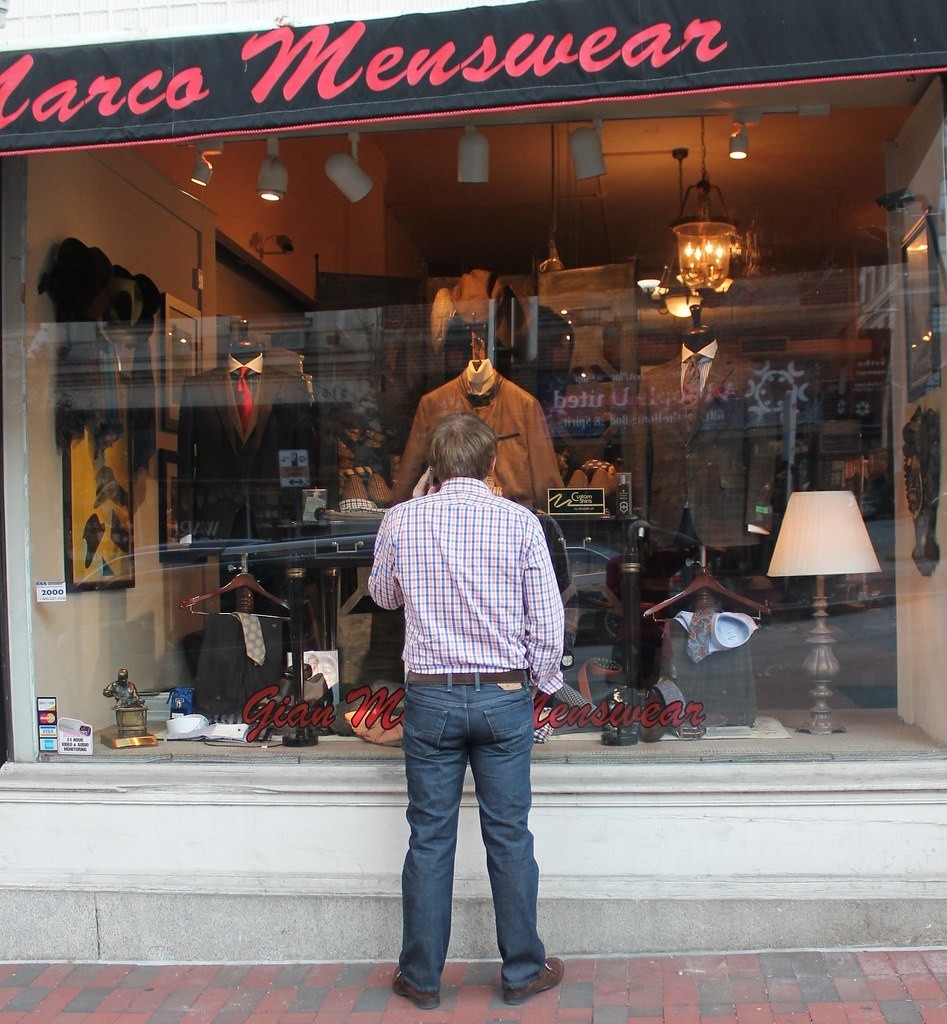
[63,373,134,594]
[162,292,203,432]
[160,449,205,564]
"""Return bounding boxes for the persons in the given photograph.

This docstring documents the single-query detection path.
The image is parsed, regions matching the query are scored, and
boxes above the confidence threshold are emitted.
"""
[178,345,312,565]
[103,669,146,708]
[632,325,779,572]
[309,655,319,671]
[515,503,578,670]
[366,411,565,1011]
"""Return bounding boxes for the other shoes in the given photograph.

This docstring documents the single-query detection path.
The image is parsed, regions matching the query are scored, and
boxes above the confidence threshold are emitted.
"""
[566,464,619,497]
[366,473,393,504]
[341,474,368,500]
[258,664,329,712]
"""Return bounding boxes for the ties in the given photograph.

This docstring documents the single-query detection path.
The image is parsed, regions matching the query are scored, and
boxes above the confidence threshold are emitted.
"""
[232,611,268,667]
[687,609,714,664]
[682,354,702,435]
[234,366,252,434]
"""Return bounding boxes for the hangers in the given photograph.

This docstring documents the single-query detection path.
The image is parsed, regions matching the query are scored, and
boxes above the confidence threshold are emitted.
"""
[179,567,291,622]
[640,568,771,621]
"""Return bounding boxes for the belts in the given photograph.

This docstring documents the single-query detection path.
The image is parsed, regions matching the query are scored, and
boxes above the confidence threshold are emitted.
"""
[578,658,623,704]
[407,669,526,684]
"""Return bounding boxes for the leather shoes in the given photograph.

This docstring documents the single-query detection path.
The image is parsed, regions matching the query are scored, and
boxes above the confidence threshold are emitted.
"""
[392,967,440,1009]
[504,957,564,1004]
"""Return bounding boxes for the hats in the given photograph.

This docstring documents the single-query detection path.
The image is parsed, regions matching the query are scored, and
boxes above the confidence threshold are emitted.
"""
[54,240,167,344]
[432,270,532,355]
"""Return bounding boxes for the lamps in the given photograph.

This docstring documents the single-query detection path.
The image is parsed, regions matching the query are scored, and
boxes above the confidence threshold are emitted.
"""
[875,188,917,214]
[729,126,749,158]
[192,154,212,186]
[260,233,294,260]
[538,122,565,273]
[257,138,287,202]
[766,491,883,736]
[456,126,490,184]
[656,119,761,295]
[636,149,738,317]
[569,119,608,181]
[325,133,373,204]
